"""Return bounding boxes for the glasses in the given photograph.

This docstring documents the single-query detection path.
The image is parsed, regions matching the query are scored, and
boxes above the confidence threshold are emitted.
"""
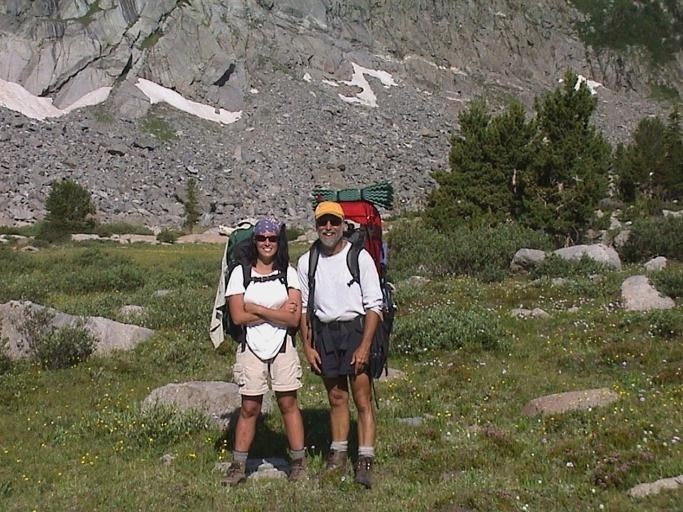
[253,234,279,242]
[316,218,342,226]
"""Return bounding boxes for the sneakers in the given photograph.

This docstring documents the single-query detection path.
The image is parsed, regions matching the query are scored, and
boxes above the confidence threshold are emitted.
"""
[318,449,348,481]
[219,459,246,486]
[352,455,374,488]
[286,455,308,482]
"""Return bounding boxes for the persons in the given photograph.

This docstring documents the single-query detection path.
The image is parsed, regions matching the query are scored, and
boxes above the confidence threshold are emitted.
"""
[222,219,306,486]
[297,202,383,488]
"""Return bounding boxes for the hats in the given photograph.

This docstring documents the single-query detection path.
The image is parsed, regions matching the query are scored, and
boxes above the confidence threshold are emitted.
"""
[314,200,345,219]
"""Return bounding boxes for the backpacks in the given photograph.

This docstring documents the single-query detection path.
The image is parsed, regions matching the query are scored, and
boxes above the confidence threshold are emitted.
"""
[224,217,291,343]
[368,283,398,380]
[305,201,385,284]
